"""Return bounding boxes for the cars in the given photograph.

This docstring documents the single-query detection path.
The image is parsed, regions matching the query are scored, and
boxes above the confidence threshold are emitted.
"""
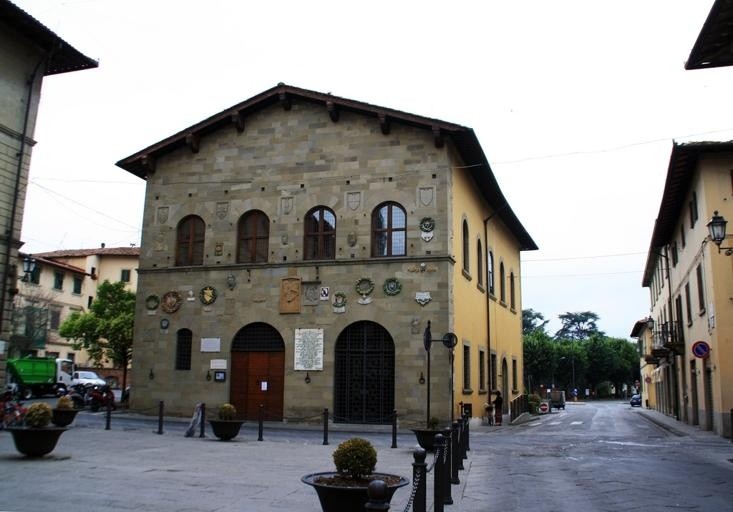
[73,371,106,385]
[630,394,641,406]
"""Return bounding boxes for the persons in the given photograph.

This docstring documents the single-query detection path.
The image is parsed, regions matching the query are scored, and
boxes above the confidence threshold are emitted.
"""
[492,390,503,426]
[591,378,640,400]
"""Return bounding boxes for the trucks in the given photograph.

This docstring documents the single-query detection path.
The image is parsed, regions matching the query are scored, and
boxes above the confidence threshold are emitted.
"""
[6,356,77,400]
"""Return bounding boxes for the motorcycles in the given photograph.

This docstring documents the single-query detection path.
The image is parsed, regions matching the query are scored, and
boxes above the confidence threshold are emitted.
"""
[68,383,115,411]
[549,391,565,410]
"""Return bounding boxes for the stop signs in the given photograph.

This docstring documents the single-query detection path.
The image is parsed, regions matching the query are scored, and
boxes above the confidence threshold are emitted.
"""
[539,402,549,414]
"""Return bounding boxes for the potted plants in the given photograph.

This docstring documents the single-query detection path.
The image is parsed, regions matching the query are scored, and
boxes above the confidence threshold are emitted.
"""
[300,438,409,512]
[49,396,79,428]
[206,403,248,441]
[411,416,452,453]
[3,403,70,457]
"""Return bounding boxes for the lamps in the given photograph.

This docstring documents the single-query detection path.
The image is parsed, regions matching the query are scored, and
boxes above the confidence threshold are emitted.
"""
[647,316,675,339]
